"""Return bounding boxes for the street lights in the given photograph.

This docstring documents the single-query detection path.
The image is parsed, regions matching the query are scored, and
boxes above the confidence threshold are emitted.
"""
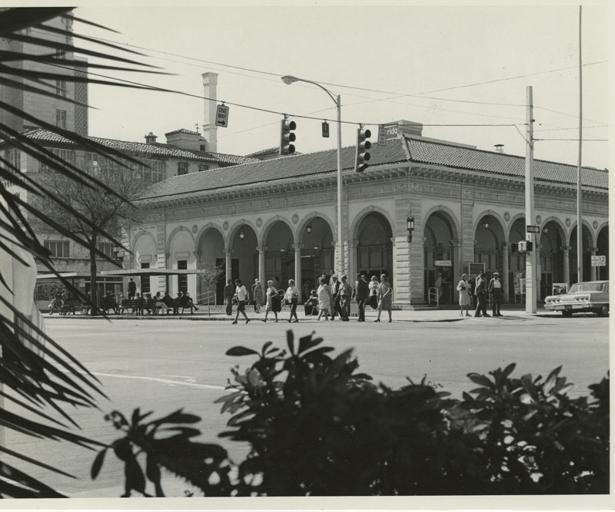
[281,75,348,279]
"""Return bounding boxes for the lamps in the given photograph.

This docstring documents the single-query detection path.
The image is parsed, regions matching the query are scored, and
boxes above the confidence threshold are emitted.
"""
[407,217,415,242]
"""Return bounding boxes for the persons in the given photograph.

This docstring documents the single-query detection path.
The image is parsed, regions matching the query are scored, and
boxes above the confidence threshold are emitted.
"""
[224,274,392,323]
[456,272,503,317]
[48,278,200,314]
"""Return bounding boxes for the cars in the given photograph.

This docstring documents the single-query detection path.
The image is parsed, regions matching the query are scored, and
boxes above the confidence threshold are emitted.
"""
[545,278,609,316]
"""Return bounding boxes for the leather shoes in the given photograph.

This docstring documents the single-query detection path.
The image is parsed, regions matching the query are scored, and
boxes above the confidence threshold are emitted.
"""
[232,318,298,324]
[316,315,391,322]
[460,312,502,316]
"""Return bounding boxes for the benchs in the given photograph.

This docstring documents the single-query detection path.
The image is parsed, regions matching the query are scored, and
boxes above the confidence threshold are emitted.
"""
[101,299,192,315]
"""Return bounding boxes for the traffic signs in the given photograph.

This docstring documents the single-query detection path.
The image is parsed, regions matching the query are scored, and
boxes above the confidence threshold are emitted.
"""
[527,225,541,233]
[214,104,229,126]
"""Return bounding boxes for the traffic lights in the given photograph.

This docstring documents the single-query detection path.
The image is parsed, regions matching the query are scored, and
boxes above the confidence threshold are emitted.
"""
[353,126,372,173]
[282,119,297,154]
